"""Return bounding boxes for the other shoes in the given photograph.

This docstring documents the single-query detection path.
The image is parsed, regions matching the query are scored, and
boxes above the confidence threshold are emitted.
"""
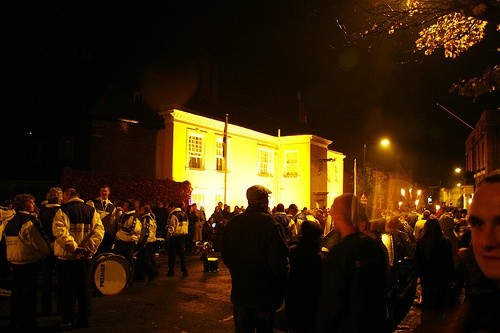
[54,321,72,330]
[74,321,89,327]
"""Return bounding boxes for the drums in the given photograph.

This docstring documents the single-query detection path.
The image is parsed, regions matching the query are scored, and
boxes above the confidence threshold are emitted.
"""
[204,257,219,272]
[90,253,132,295]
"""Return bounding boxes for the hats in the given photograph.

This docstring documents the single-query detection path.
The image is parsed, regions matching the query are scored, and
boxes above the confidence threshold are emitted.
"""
[246,184,272,199]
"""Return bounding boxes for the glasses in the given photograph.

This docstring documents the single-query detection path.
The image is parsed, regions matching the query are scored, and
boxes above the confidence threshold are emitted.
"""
[265,196,271,200]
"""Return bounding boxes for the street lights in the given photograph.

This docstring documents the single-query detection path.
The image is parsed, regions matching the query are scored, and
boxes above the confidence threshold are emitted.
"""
[368,133,392,229]
[453,166,463,174]
